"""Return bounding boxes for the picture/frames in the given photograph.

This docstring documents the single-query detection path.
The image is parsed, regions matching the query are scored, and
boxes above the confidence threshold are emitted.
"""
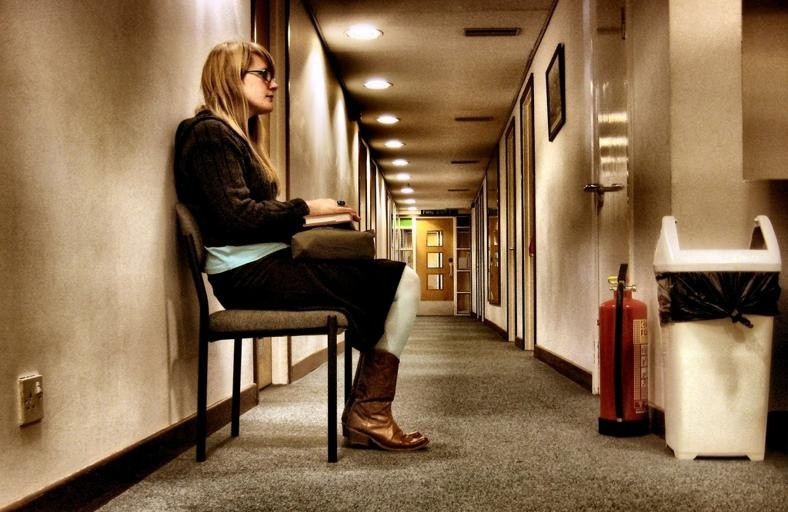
[546,42,566,143]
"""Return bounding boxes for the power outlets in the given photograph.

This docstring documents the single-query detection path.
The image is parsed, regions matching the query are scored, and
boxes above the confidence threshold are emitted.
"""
[18,375,43,427]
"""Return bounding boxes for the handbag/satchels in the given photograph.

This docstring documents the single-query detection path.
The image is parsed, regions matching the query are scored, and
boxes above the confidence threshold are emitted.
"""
[290,227,376,262]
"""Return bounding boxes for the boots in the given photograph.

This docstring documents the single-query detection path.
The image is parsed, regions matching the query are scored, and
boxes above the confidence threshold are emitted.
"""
[340,348,430,454]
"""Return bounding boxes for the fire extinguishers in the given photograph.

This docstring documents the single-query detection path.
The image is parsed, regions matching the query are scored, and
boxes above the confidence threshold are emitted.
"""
[596,263,649,439]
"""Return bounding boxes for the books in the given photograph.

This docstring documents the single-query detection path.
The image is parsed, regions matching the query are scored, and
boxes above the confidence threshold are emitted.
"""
[301,212,352,228]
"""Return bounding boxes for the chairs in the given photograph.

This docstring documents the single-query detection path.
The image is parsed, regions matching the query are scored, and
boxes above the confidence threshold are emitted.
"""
[175,206,354,462]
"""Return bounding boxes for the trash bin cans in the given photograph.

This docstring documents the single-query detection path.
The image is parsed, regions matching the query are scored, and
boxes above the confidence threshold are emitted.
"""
[652,214,782,462]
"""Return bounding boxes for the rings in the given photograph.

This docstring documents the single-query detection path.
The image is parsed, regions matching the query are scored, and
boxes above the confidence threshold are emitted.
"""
[338,201,345,205]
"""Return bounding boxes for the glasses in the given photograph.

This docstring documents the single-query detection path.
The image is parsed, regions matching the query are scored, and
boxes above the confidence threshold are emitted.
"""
[246,68,273,83]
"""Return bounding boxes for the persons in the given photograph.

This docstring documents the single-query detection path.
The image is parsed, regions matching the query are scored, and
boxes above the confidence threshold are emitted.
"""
[175,38,429,452]
